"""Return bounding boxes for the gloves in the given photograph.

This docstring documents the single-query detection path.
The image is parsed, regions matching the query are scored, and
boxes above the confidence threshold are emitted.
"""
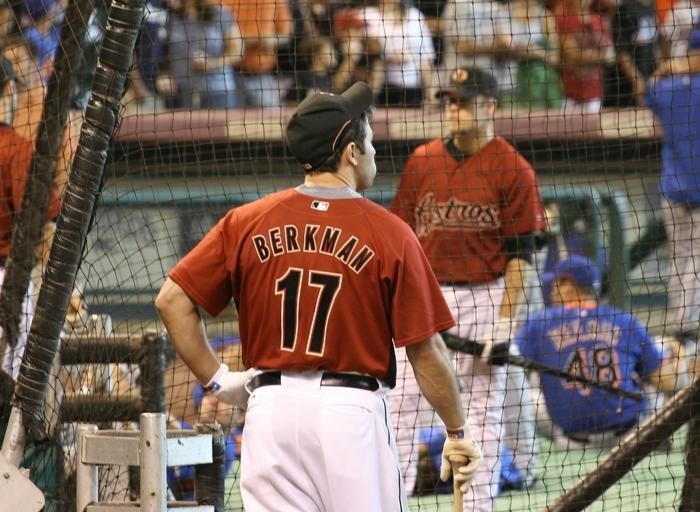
[439,424,482,494]
[203,364,256,408]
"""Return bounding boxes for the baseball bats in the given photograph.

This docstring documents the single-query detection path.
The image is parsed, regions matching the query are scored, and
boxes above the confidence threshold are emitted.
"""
[440,330,644,405]
[448,454,468,512]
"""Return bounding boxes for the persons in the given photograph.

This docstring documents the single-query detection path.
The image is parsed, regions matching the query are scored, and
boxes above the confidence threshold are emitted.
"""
[0,0,700,512]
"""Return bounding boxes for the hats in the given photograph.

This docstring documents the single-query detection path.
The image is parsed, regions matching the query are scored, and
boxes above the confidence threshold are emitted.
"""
[435,67,498,101]
[688,29,700,48]
[539,255,602,288]
[286,80,374,170]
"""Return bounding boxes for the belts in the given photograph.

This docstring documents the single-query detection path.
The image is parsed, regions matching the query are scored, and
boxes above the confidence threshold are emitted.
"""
[565,426,632,445]
[251,371,378,390]
